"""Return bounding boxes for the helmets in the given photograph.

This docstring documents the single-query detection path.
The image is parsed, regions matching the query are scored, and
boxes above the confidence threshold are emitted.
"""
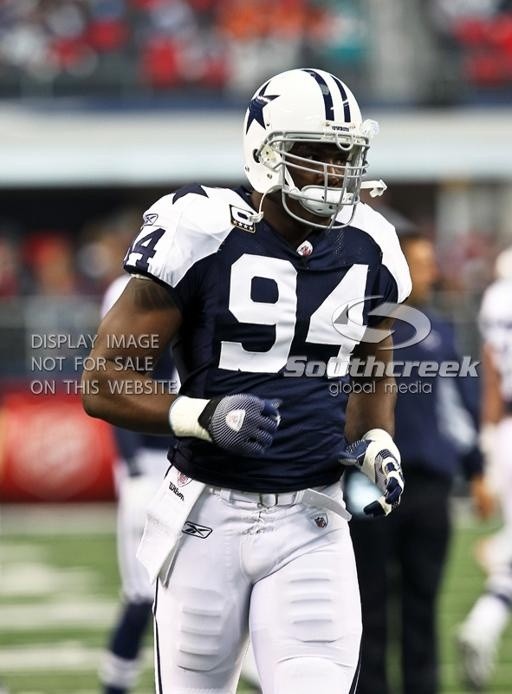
[242,67,377,228]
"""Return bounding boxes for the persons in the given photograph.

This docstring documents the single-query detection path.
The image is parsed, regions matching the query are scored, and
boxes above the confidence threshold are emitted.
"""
[78,67,405,694]
[1,1,512,384]
[349,232,482,694]
[95,275,180,694]
[459,251,512,688]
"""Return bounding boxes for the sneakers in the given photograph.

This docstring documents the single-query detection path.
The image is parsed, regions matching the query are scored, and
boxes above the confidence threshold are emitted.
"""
[457,615,500,687]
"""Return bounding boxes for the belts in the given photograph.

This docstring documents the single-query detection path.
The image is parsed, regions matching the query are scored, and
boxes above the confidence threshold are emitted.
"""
[206,485,352,525]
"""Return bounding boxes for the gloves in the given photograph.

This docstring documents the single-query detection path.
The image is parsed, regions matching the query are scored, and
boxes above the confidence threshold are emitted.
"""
[168,394,281,456]
[334,430,405,518]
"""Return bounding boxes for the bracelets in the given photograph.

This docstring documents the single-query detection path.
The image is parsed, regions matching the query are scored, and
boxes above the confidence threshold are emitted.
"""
[361,428,392,443]
[168,394,212,445]
